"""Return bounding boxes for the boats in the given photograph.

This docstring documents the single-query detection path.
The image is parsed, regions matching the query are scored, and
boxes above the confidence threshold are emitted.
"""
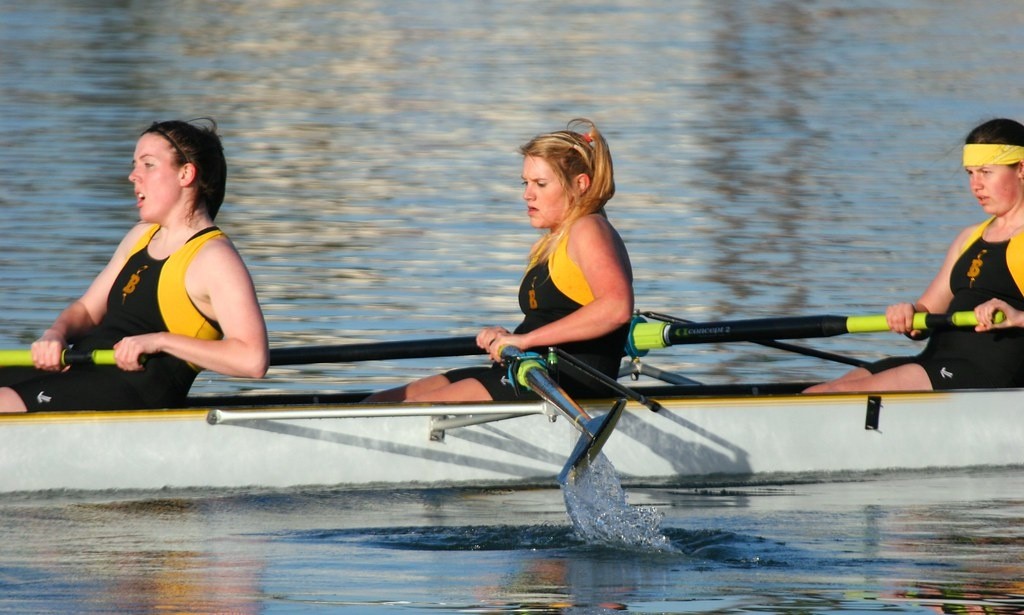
[0,384,1024,499]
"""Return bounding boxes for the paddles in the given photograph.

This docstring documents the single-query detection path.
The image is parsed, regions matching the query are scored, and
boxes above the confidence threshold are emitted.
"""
[267,308,1007,369]
[489,338,628,489]
[0,344,178,377]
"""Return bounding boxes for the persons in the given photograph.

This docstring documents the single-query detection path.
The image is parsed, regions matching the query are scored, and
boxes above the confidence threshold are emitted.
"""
[361,118,634,402]
[797,118,1024,398]
[0,115,271,413]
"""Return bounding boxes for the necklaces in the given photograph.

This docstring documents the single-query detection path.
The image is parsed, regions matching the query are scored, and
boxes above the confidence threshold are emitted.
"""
[985,224,1023,240]
